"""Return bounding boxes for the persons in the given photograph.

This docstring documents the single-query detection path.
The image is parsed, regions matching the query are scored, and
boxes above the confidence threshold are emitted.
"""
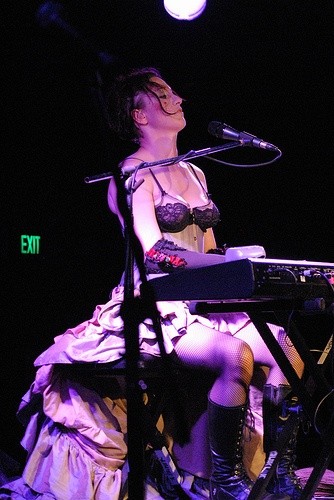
[104,66,307,500]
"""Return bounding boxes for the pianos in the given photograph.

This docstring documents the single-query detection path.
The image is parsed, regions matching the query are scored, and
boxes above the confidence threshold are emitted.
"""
[139,258,334,302]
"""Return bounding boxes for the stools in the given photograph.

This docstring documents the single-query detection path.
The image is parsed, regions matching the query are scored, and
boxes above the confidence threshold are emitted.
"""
[59,363,191,500]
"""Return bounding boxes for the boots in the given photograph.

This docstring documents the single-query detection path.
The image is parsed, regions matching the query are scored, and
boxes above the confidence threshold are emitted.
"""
[262,383,303,500]
[207,394,254,500]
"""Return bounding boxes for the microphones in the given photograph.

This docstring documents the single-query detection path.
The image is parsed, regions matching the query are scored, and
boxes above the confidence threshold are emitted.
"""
[208,120,278,152]
